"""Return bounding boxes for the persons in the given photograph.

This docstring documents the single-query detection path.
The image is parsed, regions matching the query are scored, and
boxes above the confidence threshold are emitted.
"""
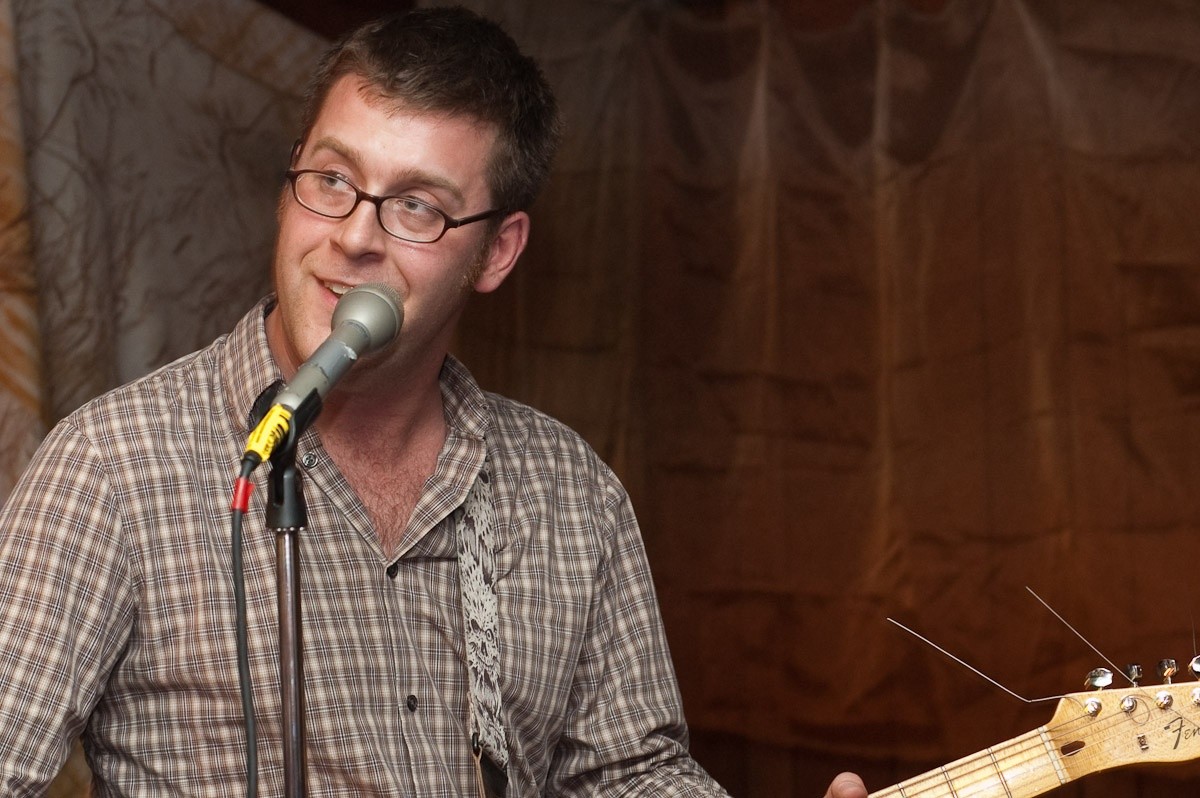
[1,0,871,798]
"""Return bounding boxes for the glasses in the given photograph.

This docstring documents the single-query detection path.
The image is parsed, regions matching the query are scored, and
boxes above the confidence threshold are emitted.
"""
[286,139,513,243]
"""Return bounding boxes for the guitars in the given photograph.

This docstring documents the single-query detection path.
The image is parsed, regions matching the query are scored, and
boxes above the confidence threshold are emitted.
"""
[864,653,1200,798]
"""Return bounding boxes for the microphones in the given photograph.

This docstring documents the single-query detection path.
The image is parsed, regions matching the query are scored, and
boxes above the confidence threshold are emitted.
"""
[252,280,405,467]
